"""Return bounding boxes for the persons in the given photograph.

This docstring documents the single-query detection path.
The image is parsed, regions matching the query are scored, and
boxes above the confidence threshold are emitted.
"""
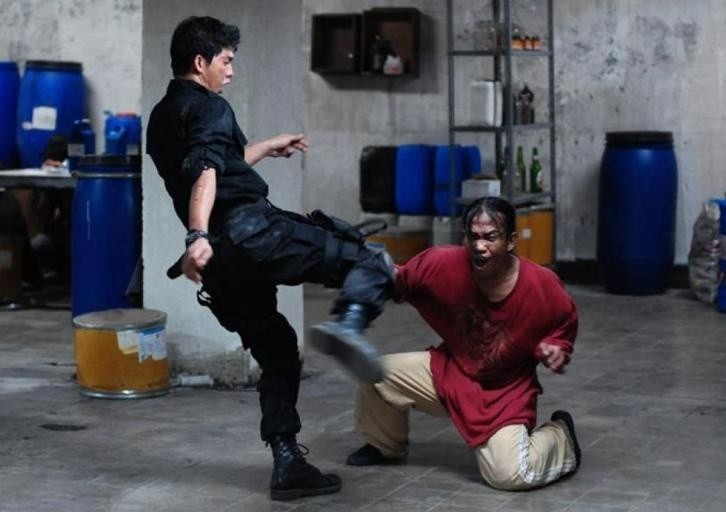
[145,13,396,502]
[344,194,582,492]
[41,152,63,169]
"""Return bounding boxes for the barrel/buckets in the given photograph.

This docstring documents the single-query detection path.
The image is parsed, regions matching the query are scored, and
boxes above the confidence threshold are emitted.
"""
[67,166,141,321]
[393,144,434,213]
[433,145,480,216]
[357,143,395,213]
[465,80,503,127]
[597,130,678,294]
[17,59,88,168]
[0,63,19,169]
[102,116,142,154]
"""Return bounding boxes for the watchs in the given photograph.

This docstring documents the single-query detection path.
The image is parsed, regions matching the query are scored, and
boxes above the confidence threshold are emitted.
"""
[184,228,209,248]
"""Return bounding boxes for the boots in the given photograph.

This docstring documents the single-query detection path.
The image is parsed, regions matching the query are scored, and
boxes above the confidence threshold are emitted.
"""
[307,304,387,385]
[270,434,342,499]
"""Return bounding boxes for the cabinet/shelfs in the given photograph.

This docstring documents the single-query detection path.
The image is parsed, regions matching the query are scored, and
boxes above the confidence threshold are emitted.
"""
[446,0,557,268]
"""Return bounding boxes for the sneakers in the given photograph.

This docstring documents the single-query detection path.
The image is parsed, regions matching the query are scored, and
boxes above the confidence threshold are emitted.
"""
[550,410,581,468]
[344,442,402,466]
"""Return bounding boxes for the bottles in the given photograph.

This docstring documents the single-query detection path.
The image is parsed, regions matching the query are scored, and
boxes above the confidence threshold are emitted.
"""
[518,82,535,123]
[514,146,527,192]
[509,26,542,52]
[528,145,544,194]
